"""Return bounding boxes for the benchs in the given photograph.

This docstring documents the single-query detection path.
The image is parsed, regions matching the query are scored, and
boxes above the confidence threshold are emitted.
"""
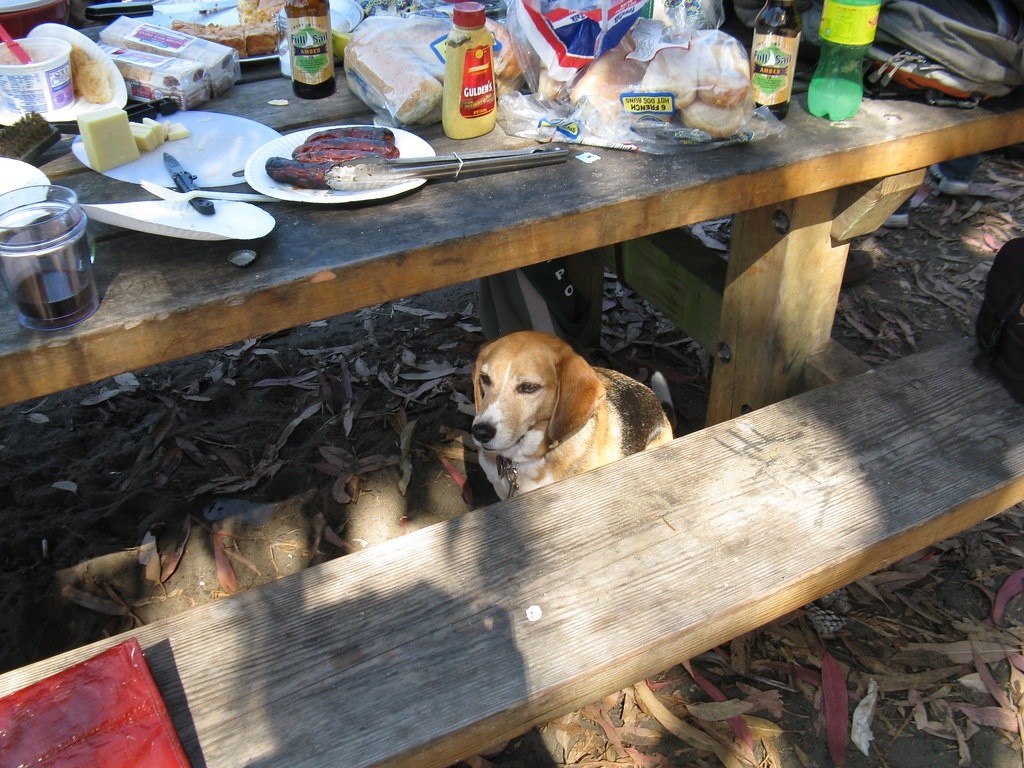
[0,338,1024,768]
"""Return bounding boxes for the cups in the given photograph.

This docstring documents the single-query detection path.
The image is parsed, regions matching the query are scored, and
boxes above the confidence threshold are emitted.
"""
[0,184,99,331]
[0,38,74,116]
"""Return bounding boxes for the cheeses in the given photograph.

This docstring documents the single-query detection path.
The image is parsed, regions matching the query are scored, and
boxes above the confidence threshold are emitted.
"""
[75,104,193,174]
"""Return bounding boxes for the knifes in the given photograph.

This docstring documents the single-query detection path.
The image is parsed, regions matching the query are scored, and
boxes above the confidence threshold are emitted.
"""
[163,151,215,215]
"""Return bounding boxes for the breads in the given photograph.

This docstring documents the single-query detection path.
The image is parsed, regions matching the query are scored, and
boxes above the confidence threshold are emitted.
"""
[347,1,761,152]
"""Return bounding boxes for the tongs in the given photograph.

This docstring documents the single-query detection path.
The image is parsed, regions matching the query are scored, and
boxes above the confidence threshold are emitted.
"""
[325,146,568,192]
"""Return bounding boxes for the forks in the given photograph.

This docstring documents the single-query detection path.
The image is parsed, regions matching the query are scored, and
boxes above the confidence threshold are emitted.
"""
[140,180,282,203]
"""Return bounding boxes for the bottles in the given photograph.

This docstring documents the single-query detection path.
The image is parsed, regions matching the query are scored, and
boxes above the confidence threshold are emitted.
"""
[749,1,803,120]
[806,0,881,120]
[442,3,496,139]
[284,1,337,100]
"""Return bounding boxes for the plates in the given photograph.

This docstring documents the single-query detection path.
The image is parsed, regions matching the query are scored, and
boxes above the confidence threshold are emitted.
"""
[246,125,435,203]
[0,156,50,233]
[77,201,274,241]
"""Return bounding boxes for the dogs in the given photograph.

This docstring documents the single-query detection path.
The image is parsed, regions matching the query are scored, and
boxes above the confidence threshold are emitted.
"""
[470,330,678,503]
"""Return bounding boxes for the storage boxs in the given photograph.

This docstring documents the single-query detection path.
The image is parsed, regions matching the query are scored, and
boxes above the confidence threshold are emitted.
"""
[93,14,241,112]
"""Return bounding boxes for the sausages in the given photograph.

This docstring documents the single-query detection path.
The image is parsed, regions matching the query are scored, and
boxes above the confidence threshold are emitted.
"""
[266,125,398,190]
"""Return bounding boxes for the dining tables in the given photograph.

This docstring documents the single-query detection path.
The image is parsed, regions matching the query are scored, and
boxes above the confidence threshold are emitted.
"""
[0,0,1024,406]
